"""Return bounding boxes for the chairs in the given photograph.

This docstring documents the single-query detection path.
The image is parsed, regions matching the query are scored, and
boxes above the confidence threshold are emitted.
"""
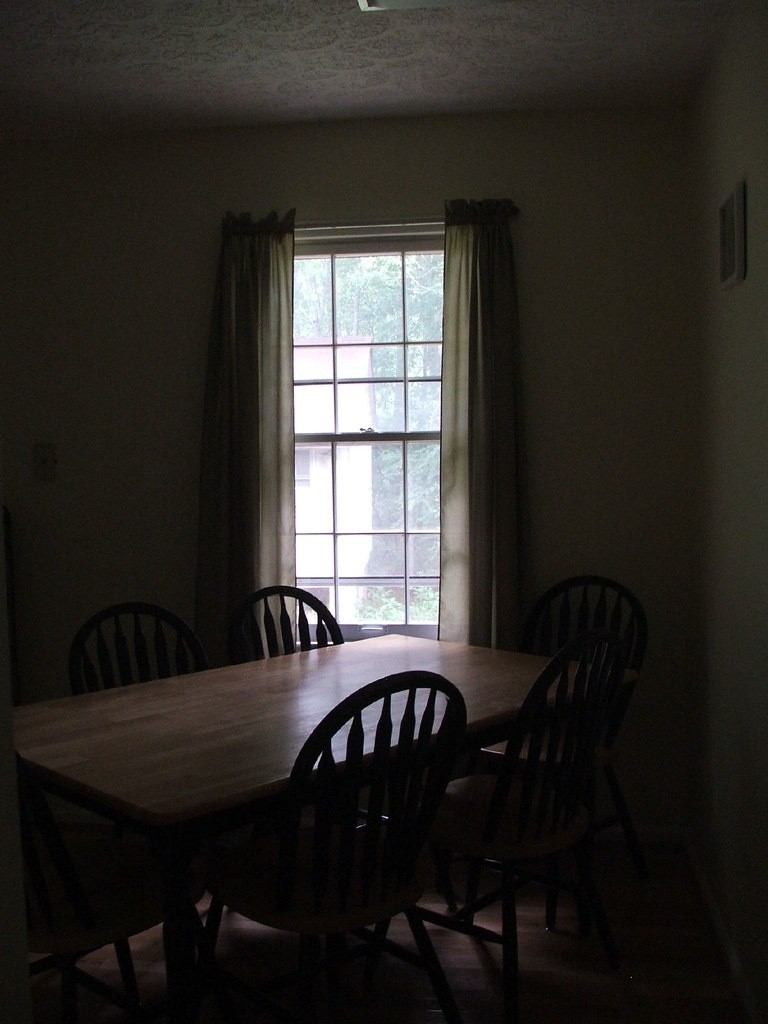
[69,602,211,696]
[482,575,648,877]
[188,670,467,1024]
[430,627,619,1024]
[16,780,238,1024]
[228,585,457,912]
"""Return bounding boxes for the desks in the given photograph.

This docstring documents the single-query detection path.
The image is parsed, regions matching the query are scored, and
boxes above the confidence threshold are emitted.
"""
[11,633,639,1024]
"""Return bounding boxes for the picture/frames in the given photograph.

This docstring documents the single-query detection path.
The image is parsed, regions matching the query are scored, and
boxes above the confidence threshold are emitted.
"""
[717,182,744,291]
